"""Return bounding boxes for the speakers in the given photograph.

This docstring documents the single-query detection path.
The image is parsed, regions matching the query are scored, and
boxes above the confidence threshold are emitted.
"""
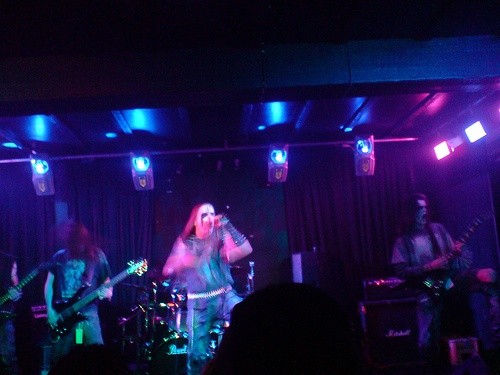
[357,295,420,369]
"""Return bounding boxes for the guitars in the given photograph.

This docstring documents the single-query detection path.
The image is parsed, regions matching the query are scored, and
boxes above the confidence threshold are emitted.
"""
[406,207,494,312]
[47,258,148,339]
[0,266,43,323]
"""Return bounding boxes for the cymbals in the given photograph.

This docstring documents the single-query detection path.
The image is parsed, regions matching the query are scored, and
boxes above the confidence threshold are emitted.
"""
[228,264,249,274]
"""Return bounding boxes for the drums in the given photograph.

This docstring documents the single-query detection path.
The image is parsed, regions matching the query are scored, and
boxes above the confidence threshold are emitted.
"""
[145,320,213,375]
[135,303,168,348]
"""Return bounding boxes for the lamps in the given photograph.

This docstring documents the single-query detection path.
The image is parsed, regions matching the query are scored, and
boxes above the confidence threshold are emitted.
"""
[354,133,375,176]
[129,151,153,191]
[266,143,289,184]
[30,154,55,196]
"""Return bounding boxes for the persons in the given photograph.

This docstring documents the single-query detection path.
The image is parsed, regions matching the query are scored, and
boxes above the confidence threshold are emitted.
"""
[41,220,113,375]
[0,251,22,375]
[162,202,253,375]
[391,193,500,375]
